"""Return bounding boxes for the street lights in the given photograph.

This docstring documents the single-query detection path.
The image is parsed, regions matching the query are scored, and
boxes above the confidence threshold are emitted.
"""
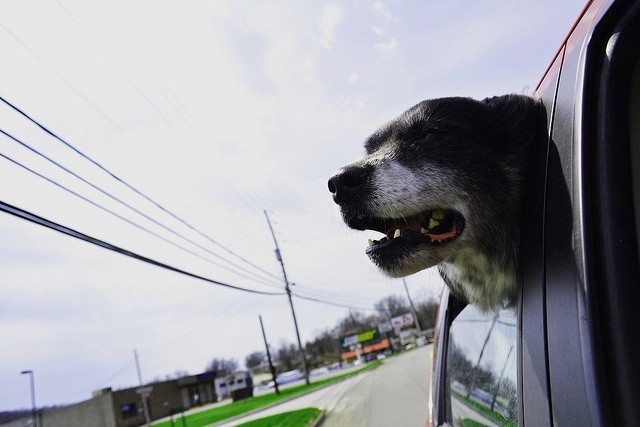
[21,370,36,427]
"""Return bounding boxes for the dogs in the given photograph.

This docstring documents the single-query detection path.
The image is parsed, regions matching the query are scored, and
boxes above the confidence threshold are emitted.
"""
[328,93,547,317]
